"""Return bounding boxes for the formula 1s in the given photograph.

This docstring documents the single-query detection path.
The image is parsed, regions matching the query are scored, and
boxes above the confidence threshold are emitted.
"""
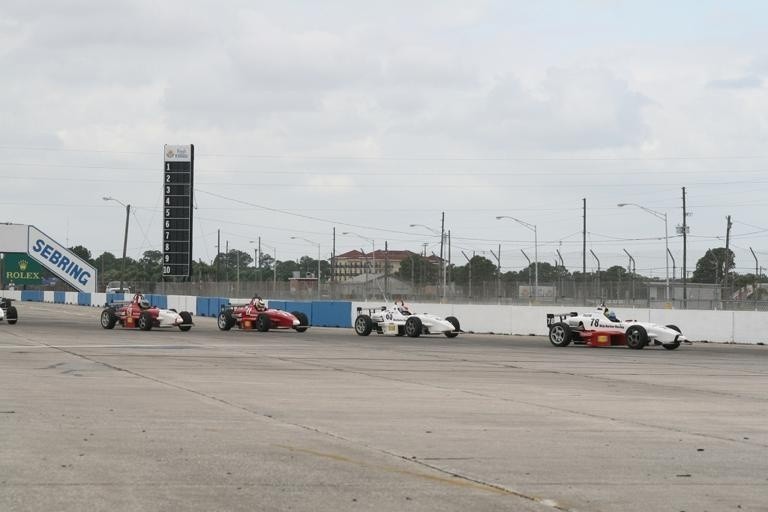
[355,299,464,337]
[546,302,693,350]
[217,294,310,332]
[101,291,195,330]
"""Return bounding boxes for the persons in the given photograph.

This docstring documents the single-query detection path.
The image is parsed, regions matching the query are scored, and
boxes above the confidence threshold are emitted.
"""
[132,290,144,304]
[607,310,617,321]
[254,301,268,312]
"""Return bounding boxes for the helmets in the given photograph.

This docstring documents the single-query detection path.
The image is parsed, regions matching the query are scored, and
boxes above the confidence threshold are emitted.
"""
[255,300,266,311]
[605,310,616,322]
[399,305,409,316]
[140,299,151,308]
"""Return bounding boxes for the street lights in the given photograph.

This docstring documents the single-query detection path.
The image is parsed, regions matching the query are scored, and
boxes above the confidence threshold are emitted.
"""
[249,239,277,293]
[214,244,241,298]
[342,231,376,297]
[409,224,447,298]
[103,196,130,293]
[496,216,539,297]
[290,236,322,297]
[618,203,670,301]
